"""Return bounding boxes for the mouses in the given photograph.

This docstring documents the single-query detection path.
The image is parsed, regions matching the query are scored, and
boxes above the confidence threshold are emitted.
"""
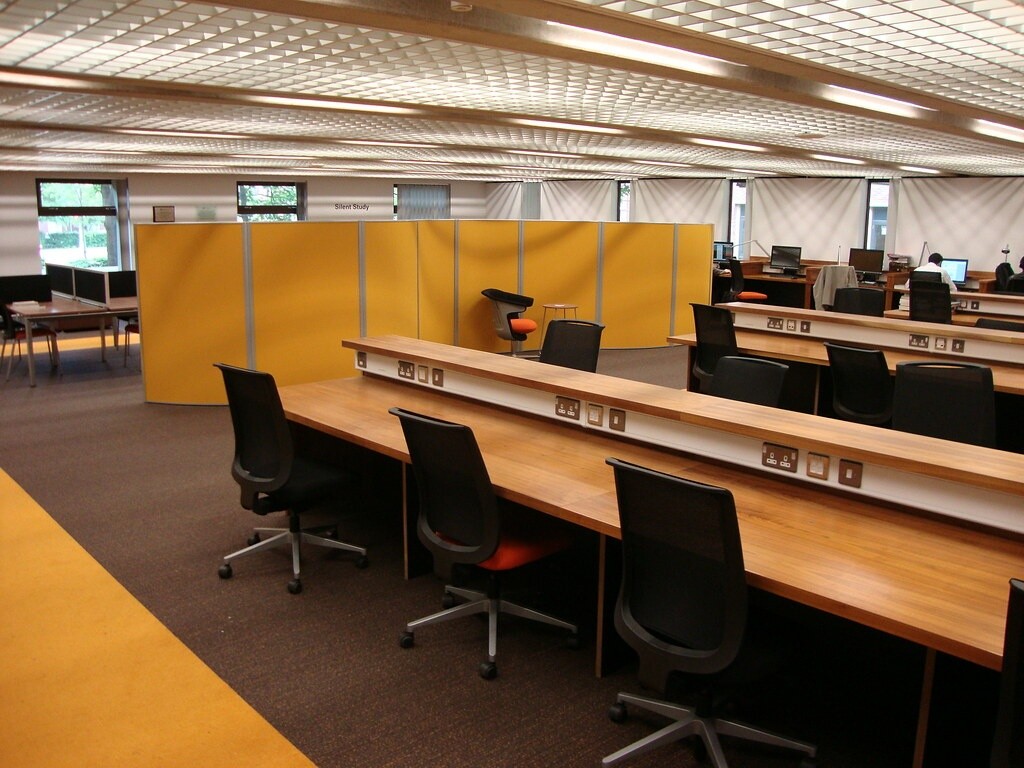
[792,276,797,279]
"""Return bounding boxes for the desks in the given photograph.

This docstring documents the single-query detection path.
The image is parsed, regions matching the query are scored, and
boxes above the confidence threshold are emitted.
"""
[561,466,1024,768]
[538,304,577,355]
[6,294,107,387]
[718,254,885,312]
[269,375,695,681]
[884,309,1024,327]
[105,297,140,349]
[666,329,829,415]
[1,471,313,767]
[877,350,1024,396]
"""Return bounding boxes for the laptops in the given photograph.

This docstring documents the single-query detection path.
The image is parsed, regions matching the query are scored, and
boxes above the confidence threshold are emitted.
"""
[940,259,968,285]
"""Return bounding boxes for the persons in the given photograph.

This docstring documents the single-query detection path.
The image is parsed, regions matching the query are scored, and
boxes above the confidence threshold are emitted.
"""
[1006,256,1024,292]
[905,252,957,291]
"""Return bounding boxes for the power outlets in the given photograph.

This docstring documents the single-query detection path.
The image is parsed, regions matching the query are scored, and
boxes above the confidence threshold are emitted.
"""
[398,360,416,379]
[767,318,783,330]
[909,333,930,349]
[555,396,581,422]
[761,442,799,473]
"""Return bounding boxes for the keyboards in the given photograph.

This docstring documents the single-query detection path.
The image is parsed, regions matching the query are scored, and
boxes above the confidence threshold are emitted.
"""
[769,274,806,278]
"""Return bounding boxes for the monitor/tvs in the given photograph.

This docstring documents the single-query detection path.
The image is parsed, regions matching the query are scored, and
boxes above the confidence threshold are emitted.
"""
[713,242,734,262]
[770,246,801,275]
[848,248,884,281]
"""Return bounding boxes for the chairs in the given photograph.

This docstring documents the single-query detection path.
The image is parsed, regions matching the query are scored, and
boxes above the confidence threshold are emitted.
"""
[0,259,1024,768]
